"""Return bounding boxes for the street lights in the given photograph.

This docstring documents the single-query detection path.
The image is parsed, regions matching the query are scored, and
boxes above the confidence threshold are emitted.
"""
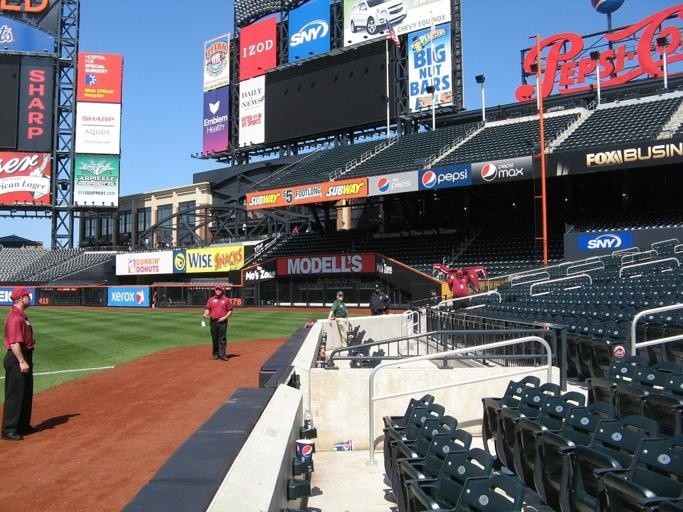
[528,34,548,267]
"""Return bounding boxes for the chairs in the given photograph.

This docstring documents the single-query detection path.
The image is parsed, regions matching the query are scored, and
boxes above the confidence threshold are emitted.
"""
[258,88,683,192]
[264,225,683,511]
[0,247,108,285]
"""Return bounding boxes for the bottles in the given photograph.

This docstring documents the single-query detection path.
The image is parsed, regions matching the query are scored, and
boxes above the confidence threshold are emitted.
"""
[321,332,325,343]
[304,409,312,431]
[320,348,325,357]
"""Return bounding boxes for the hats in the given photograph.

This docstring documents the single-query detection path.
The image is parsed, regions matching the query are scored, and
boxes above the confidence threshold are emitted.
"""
[375,284,381,289]
[11,288,33,300]
[215,285,223,291]
[337,291,344,296]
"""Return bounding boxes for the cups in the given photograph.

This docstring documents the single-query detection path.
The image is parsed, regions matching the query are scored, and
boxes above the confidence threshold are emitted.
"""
[316,361,323,368]
[297,439,313,463]
[333,440,353,451]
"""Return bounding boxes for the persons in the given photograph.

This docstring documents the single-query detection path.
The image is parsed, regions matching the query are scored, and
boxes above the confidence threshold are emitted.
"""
[203,284,233,361]
[432,263,486,308]
[2,287,42,440]
[327,290,349,320]
[150,290,159,310]
[368,283,391,317]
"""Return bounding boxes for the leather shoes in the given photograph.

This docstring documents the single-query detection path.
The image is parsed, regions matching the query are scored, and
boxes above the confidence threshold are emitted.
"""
[7,432,23,439]
[213,353,228,360]
